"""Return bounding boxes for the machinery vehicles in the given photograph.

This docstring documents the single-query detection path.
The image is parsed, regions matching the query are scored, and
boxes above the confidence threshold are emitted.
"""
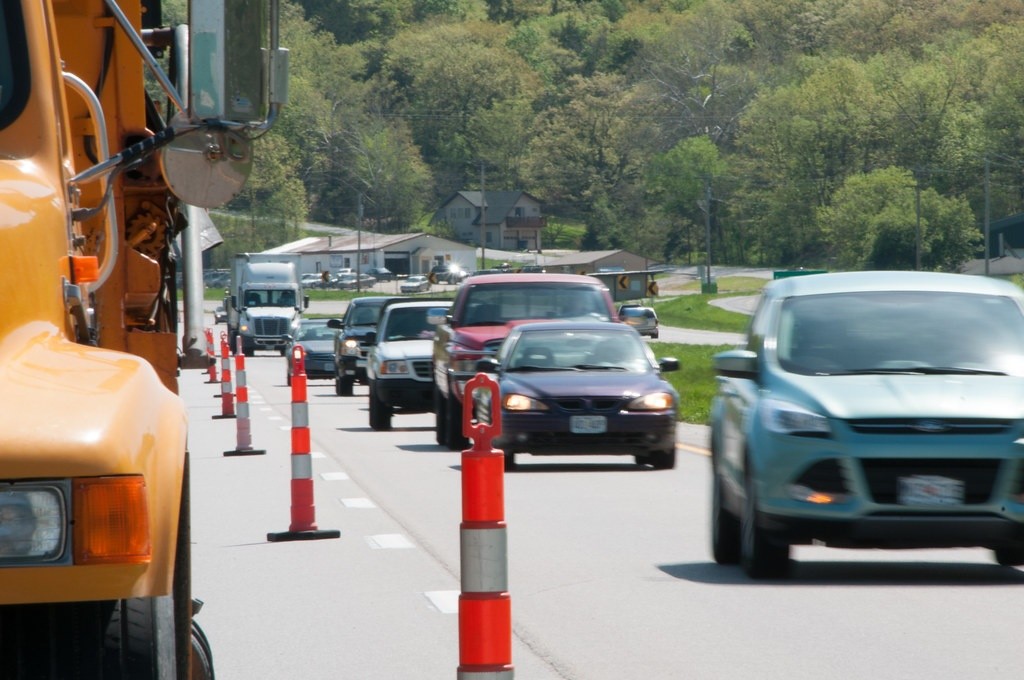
[1,0,306,680]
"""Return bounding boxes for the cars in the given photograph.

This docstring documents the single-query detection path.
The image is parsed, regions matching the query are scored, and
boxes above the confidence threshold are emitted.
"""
[177,264,546,293]
[369,300,463,429]
[214,307,228,325]
[428,273,622,447]
[499,320,684,472]
[328,296,417,397]
[284,320,344,387]
[710,268,1024,586]
[618,303,659,339]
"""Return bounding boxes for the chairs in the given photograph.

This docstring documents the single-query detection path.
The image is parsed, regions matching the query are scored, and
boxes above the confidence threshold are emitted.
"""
[520,348,554,366]
[472,305,500,323]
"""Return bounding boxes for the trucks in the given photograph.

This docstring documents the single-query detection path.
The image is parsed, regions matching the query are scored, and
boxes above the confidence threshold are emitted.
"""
[224,252,302,358]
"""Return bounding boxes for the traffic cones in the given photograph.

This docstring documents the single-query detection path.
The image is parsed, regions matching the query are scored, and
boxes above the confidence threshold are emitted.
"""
[267,347,340,544]
[455,376,517,680]
[202,327,238,420]
[223,336,267,457]
